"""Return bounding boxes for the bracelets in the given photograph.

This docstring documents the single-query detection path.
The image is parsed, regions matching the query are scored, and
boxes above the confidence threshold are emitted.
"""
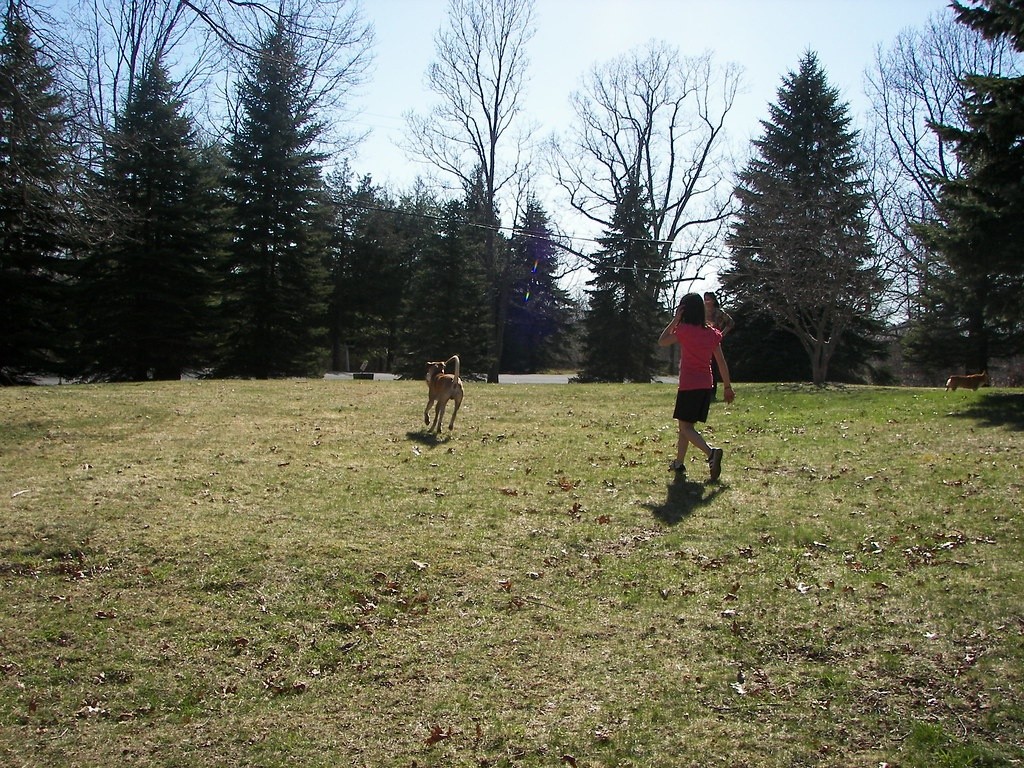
[725,386,731,390]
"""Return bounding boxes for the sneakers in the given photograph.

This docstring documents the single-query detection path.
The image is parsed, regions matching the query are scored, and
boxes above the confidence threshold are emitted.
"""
[706,449,723,480]
[669,460,686,473]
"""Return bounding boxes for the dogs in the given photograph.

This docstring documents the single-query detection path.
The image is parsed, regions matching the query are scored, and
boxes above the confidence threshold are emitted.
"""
[944,370,989,392]
[424,354,464,435]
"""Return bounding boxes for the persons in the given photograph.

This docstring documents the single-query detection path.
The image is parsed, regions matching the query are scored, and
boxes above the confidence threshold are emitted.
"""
[658,293,735,482]
[704,291,734,401]
